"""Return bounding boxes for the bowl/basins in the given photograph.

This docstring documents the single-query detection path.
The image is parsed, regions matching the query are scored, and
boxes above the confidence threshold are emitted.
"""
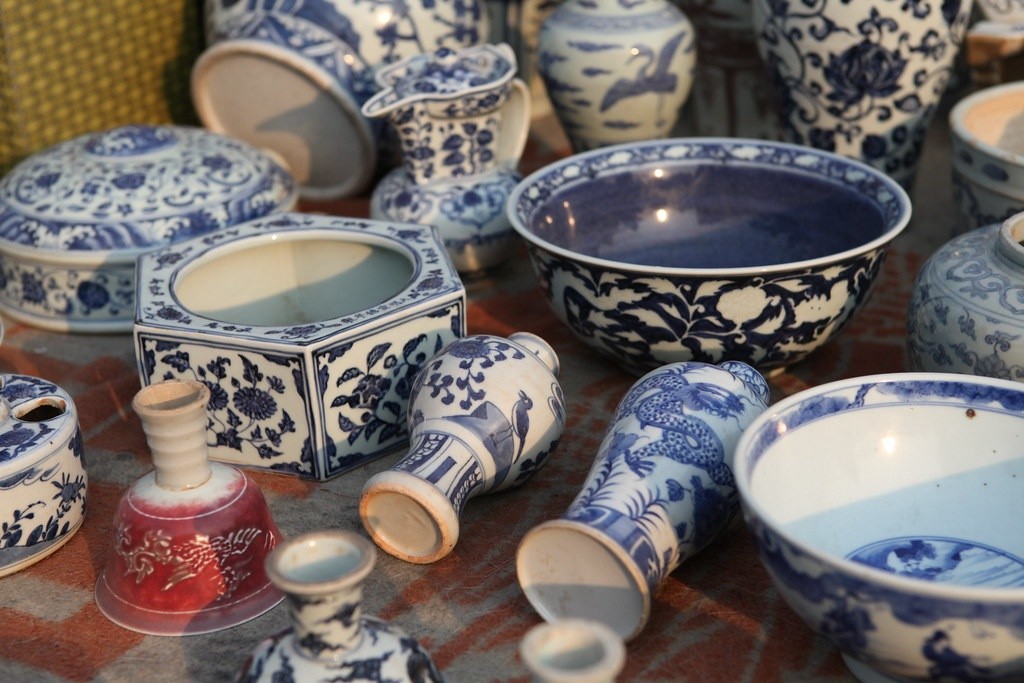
[503,139,911,376]
[733,371,1024,683]
[949,82,1024,231]
